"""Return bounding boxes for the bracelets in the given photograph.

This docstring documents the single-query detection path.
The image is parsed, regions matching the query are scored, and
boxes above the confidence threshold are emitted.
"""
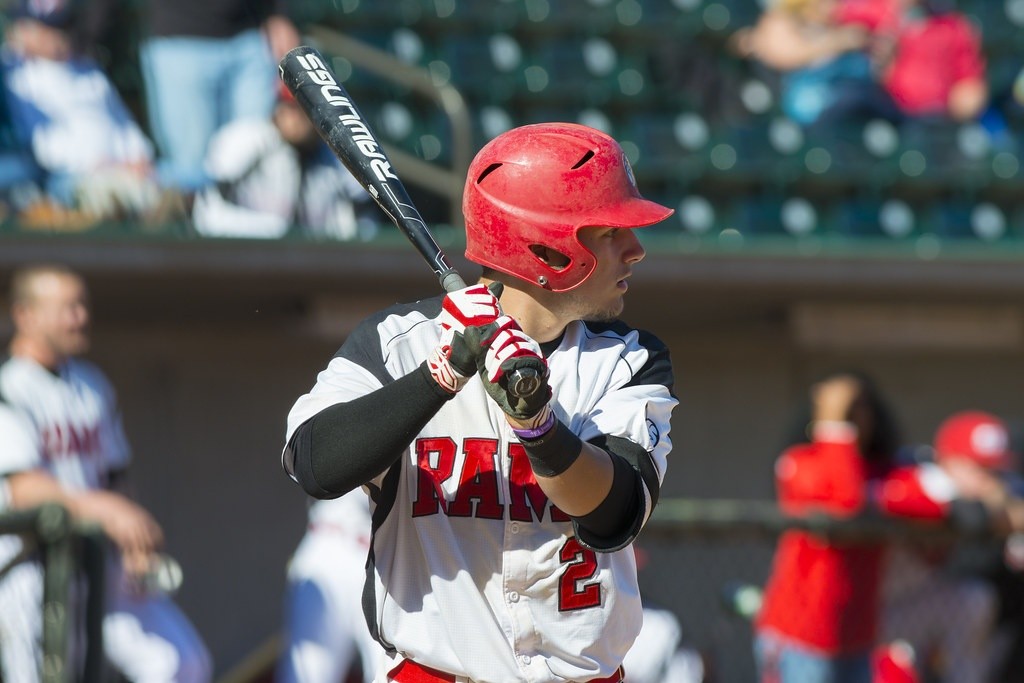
[511,411,555,438]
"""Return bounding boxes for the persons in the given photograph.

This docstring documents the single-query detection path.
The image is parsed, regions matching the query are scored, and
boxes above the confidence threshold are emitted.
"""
[285,123,678,683]
[0,0,377,244]
[0,265,212,683]
[279,485,404,683]
[749,368,1024,683]
[737,0,1010,142]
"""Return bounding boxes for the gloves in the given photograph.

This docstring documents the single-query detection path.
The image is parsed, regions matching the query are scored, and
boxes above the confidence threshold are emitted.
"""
[425,281,506,396]
[455,313,558,440]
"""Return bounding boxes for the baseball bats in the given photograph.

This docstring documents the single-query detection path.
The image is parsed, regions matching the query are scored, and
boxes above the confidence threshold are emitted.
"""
[276,42,545,399]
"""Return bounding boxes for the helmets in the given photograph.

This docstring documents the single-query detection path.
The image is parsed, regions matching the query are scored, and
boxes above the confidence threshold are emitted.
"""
[940,413,1018,470]
[459,123,674,294]
